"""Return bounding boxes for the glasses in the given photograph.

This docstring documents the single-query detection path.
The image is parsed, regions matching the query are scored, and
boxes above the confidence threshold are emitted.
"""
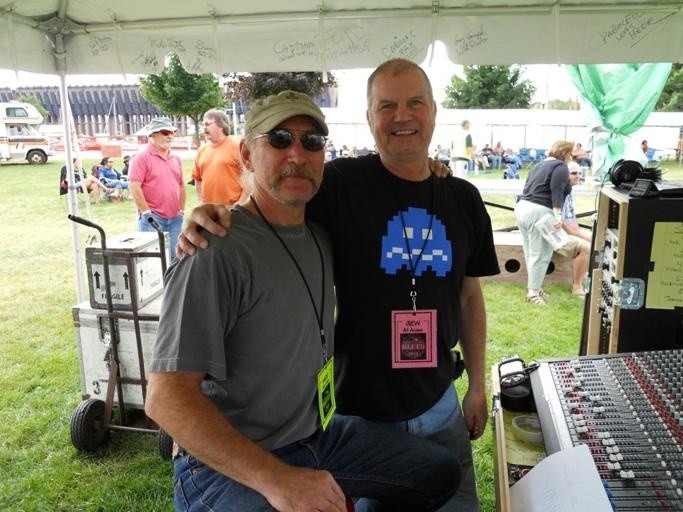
[252,129,329,151]
[202,120,216,127]
[160,131,169,136]
[570,171,581,175]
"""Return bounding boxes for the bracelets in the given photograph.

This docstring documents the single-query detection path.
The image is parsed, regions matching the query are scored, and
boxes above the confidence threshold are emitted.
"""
[142,210,151,216]
[178,209,183,212]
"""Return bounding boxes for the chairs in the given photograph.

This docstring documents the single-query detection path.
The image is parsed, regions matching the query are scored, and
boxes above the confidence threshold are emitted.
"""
[646,148,657,168]
[60,166,96,208]
[91,163,128,201]
[534,149,547,162]
[520,149,535,169]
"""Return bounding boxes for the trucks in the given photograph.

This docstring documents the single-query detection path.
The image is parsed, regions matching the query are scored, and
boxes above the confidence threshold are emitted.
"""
[0,101,53,163]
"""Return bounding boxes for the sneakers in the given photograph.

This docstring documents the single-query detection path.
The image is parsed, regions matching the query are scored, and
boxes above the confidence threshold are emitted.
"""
[539,289,549,296]
[524,295,545,306]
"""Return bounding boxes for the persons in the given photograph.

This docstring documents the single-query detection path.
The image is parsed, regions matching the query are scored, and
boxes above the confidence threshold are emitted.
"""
[65,158,115,206]
[560,161,593,300]
[495,142,503,155]
[98,157,134,199]
[144,90,461,512]
[122,155,131,175]
[642,140,649,154]
[473,146,490,172]
[514,142,574,307]
[461,120,474,160]
[324,141,380,160]
[176,58,500,512]
[191,108,253,210]
[128,117,185,261]
[572,143,592,167]
[482,144,502,169]
[434,145,450,166]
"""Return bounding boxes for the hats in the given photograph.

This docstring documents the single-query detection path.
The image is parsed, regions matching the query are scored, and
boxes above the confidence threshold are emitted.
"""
[243,90,329,144]
[123,155,131,163]
[146,117,179,138]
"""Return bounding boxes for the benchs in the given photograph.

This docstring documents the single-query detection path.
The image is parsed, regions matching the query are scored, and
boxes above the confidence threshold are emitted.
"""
[483,232,574,284]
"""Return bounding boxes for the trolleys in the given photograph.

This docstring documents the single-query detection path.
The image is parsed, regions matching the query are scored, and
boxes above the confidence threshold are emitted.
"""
[57,209,180,460]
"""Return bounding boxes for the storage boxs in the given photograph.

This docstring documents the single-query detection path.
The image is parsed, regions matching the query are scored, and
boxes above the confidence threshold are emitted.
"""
[85,231,171,311]
[71,294,162,410]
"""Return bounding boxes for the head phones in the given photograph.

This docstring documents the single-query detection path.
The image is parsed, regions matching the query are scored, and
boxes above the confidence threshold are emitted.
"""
[608,159,662,187]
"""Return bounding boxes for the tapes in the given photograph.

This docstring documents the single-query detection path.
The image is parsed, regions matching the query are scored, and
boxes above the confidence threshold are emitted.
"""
[511,415,544,443]
